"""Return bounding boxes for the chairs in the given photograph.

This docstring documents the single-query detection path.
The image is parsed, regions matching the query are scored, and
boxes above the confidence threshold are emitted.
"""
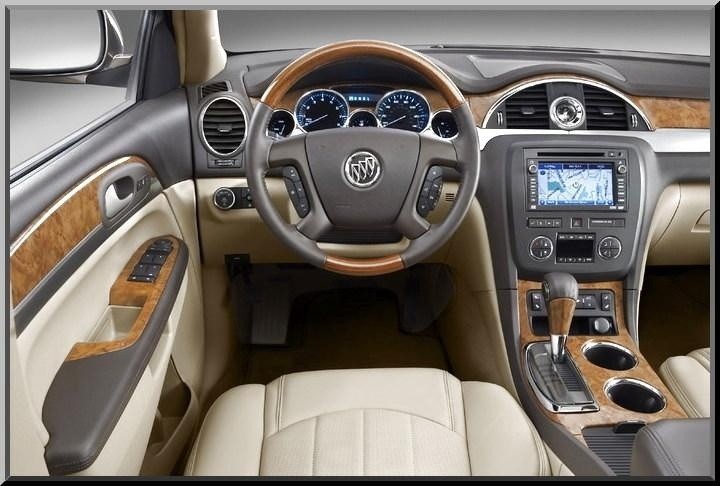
[182,364,552,477]
